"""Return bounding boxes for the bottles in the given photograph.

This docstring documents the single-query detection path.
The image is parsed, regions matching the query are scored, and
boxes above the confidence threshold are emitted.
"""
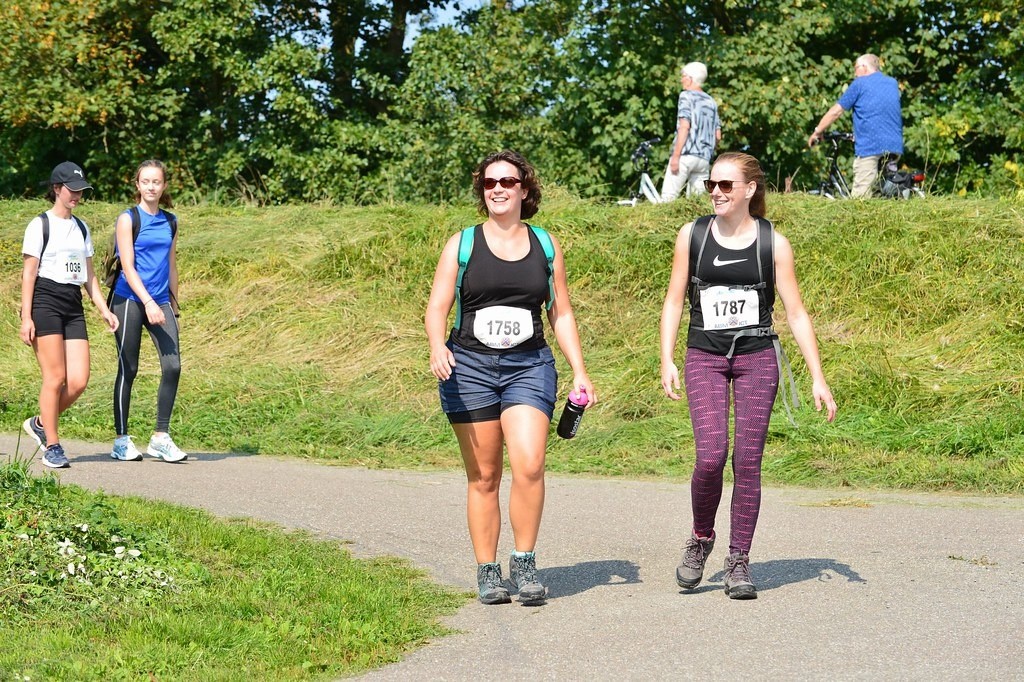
[557,387,587,439]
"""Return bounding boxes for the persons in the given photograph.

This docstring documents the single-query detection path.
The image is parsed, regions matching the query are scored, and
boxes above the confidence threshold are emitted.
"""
[809,55,904,200]
[425,150,598,606]
[660,62,722,204]
[18,161,120,468]
[660,153,838,599]
[106,160,189,463]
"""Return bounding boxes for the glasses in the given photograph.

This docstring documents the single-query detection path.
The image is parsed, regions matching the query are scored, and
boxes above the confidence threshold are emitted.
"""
[483,177,522,189]
[703,180,749,193]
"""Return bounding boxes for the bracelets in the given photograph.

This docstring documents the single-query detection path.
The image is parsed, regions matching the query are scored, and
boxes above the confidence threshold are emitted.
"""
[815,127,821,134]
[144,299,153,306]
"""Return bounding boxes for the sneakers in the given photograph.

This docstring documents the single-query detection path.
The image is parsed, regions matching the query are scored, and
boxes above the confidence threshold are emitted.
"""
[42,443,69,468]
[724,548,757,600]
[146,433,188,462]
[477,563,511,604]
[508,553,545,601]
[676,530,715,588]
[111,436,143,461]
[22,415,47,451]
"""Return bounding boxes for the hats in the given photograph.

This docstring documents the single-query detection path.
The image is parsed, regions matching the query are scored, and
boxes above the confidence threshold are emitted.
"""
[51,161,94,191]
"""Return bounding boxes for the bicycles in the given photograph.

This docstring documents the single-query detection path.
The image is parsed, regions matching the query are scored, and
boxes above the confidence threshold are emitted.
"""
[614,138,712,206]
[804,131,927,201]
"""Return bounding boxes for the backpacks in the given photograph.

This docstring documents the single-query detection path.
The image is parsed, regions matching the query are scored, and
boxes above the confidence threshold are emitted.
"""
[104,206,174,287]
